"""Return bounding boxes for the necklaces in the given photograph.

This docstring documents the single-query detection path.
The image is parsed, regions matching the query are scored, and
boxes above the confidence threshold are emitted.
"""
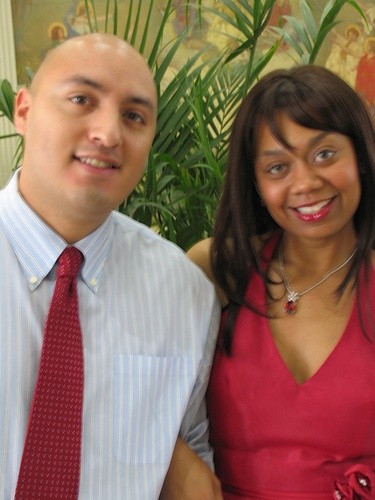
[278,247,359,312]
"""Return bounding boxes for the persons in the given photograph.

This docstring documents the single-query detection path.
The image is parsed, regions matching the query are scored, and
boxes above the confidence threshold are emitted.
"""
[1,33,221,500]
[158,65,375,500]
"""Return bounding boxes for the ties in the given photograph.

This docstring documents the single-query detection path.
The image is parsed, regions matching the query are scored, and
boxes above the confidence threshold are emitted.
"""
[15,247,86,500]
